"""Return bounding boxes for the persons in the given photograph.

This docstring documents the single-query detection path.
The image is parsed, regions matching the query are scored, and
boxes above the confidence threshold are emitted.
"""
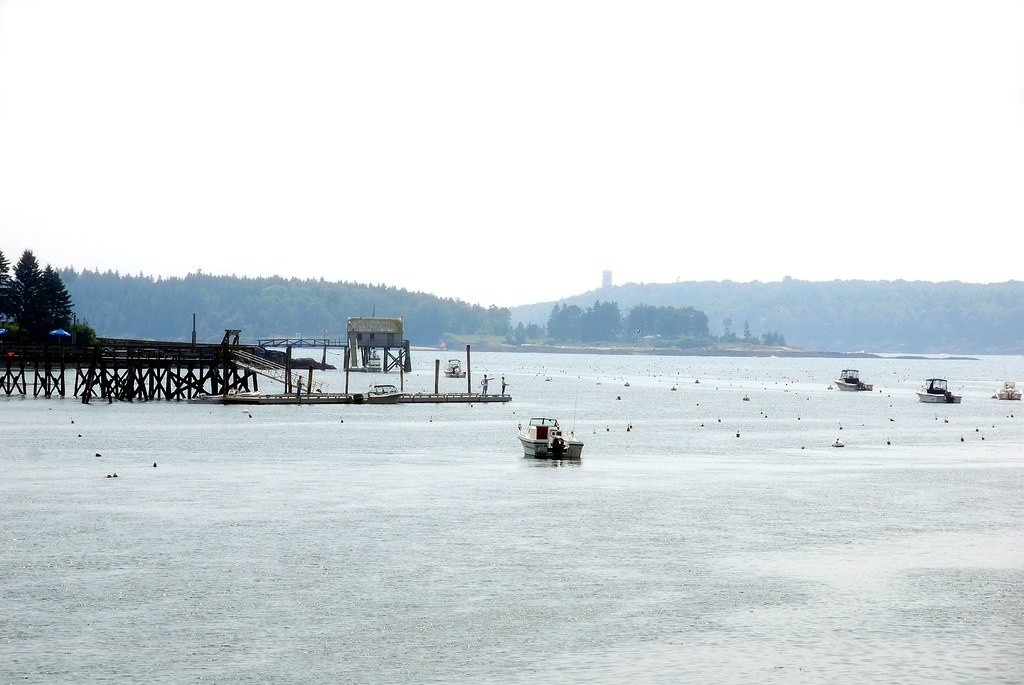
[502,377,509,397]
[481,375,492,397]
[297,376,305,398]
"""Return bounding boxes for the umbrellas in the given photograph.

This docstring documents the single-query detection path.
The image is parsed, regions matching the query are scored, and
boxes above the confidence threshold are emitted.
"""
[49,328,71,351]
[0,329,8,335]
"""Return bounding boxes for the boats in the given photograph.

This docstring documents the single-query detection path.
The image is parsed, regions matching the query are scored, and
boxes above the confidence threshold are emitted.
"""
[443,358,466,378]
[366,357,382,372]
[833,369,874,393]
[997,381,1023,400]
[517,417,585,459]
[915,378,962,404]
[348,383,408,404]
